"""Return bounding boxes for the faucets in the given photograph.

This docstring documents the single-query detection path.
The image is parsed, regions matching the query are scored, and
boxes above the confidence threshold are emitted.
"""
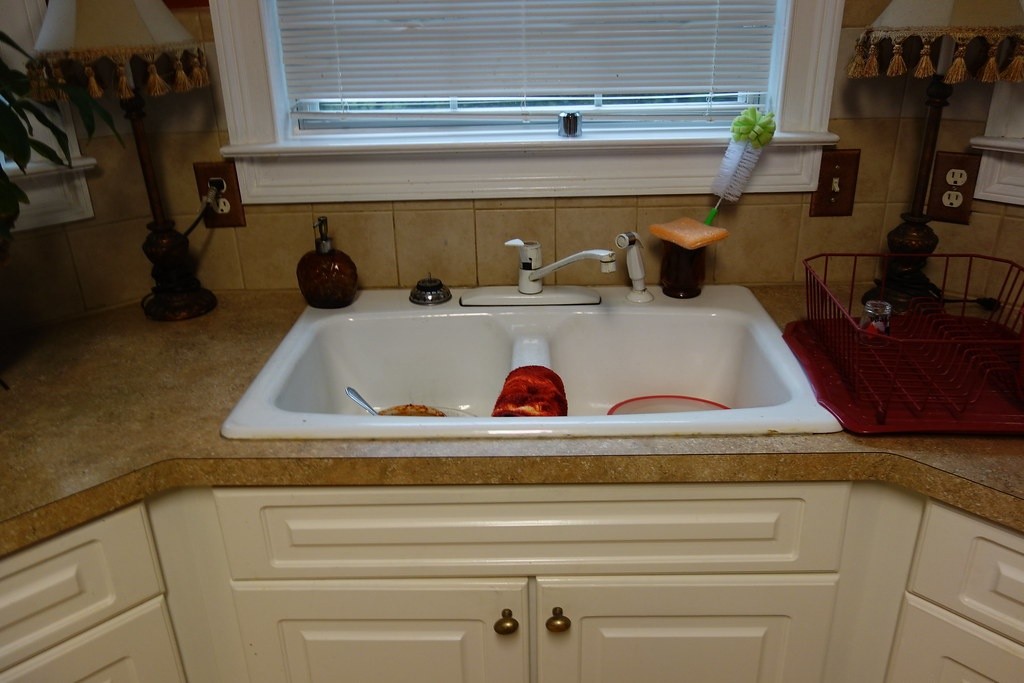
[503,237,617,294]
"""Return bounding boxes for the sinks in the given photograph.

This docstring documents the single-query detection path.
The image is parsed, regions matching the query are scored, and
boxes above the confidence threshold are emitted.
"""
[220,282,846,440]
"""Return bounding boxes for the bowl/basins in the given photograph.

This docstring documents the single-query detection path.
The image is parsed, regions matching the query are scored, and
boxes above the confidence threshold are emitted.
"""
[607,396,730,416]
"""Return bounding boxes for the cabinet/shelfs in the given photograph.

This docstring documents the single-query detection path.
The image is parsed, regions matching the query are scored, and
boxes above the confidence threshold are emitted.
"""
[0,481,1024,683]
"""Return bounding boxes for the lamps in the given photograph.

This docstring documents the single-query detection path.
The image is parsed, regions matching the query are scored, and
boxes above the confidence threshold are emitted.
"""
[848,0,1024,321]
[30,0,218,322]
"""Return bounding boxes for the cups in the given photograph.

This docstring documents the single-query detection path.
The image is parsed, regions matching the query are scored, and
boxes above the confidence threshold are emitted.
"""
[856,300,891,347]
[660,240,706,299]
[378,404,447,416]
[558,110,583,137]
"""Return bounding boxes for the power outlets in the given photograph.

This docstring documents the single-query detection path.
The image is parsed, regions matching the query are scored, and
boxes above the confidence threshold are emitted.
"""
[926,150,982,226]
[193,161,247,229]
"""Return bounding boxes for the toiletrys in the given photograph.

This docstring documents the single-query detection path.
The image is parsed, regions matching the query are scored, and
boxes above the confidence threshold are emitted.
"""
[296,216,357,309]
